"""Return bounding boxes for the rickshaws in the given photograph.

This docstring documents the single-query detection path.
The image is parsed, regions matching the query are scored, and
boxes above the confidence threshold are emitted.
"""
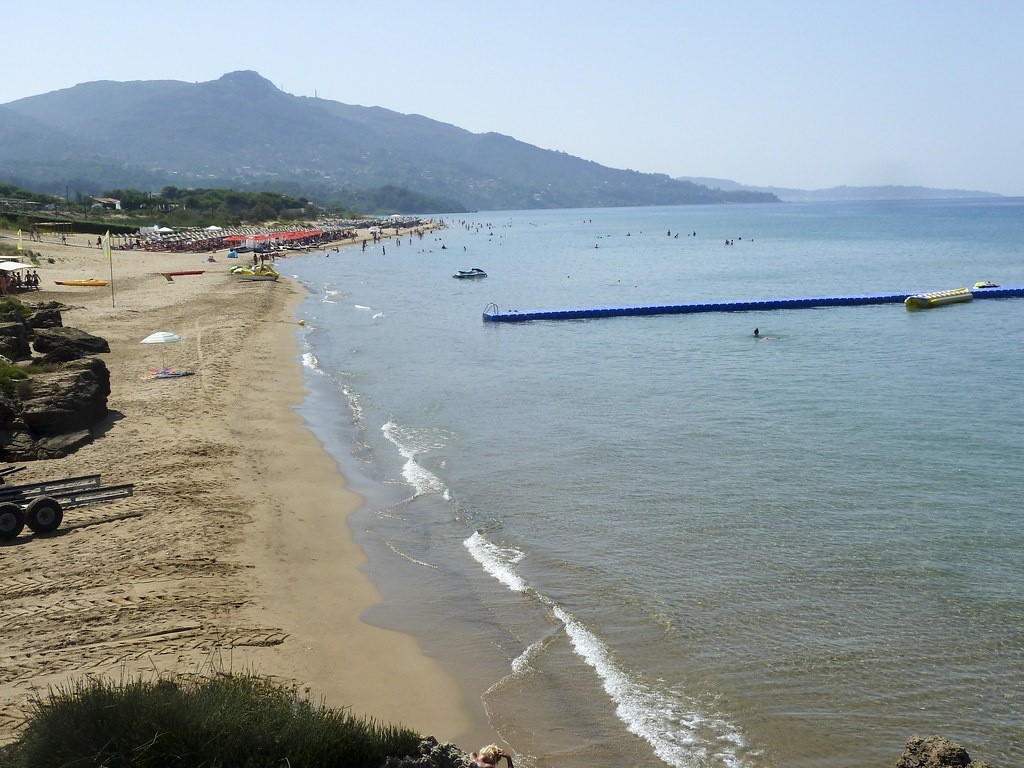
[0,474,135,540]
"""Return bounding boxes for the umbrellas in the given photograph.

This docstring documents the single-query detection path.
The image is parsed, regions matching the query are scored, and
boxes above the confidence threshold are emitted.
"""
[140,332,180,370]
[110,224,324,244]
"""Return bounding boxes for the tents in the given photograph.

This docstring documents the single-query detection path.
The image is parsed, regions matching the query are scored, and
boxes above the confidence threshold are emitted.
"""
[0,261,33,272]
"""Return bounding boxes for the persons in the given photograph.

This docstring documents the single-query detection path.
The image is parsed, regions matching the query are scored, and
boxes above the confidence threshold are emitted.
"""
[61,233,67,245]
[753,328,760,337]
[229,218,592,266]
[87,240,92,248]
[29,229,36,242]
[0,270,42,296]
[36,230,42,242]
[593,228,754,249]
[96,236,102,249]
[113,236,240,254]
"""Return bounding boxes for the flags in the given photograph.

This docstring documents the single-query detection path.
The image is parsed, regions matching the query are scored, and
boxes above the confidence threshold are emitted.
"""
[100,229,111,258]
[16,229,23,250]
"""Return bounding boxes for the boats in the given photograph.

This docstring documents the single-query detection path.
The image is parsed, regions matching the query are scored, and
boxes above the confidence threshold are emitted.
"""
[973,280,1003,289]
[904,287,973,308]
[54,279,109,286]
[453,267,487,280]
[230,263,279,281]
